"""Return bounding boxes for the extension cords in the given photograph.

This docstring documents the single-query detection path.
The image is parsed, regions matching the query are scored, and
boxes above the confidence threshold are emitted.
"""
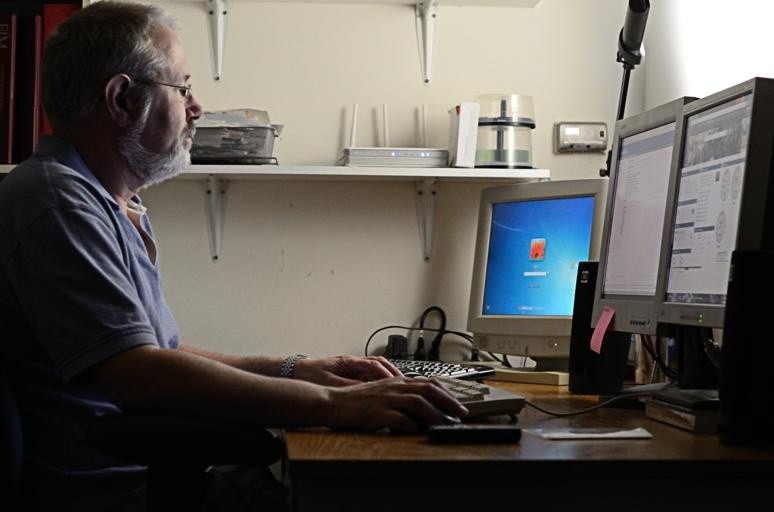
[455,360,502,367]
[438,358,502,370]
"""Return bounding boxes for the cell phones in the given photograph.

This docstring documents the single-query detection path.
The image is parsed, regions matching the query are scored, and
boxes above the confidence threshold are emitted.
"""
[427,420,522,446]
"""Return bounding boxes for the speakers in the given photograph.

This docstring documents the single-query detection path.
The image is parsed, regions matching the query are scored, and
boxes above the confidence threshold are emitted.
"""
[568,261,633,397]
[719,248,774,453]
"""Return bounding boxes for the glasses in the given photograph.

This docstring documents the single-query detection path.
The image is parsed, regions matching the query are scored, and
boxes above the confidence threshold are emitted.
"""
[136,76,192,98]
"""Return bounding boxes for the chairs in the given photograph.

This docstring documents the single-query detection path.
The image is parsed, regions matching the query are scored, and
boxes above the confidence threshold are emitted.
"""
[0,352,284,512]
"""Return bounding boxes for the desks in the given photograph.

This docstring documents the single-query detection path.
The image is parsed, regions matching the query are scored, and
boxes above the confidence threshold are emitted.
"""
[281,370,774,512]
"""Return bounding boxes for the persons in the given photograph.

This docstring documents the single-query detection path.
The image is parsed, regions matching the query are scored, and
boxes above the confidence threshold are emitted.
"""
[0,1,473,511]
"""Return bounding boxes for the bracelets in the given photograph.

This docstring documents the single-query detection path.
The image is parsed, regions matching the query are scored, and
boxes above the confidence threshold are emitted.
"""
[276,352,310,377]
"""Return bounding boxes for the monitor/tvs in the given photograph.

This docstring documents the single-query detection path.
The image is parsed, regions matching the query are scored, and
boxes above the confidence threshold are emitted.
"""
[466,176,609,388]
[655,76,774,411]
[591,94,700,412]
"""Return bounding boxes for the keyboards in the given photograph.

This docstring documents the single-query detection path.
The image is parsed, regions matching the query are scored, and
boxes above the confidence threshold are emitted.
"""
[385,356,496,390]
[425,374,527,420]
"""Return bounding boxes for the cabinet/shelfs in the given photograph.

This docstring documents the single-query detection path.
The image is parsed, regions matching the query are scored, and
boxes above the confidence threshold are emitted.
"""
[0,0,552,261]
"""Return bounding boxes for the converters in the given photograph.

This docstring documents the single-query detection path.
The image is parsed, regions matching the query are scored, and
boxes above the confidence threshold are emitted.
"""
[386,335,410,358]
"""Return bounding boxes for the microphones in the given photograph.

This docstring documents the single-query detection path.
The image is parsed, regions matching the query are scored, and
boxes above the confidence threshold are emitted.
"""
[613,1,651,65]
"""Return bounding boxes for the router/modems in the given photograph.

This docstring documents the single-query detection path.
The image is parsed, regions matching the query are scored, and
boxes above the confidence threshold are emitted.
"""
[342,103,449,167]
